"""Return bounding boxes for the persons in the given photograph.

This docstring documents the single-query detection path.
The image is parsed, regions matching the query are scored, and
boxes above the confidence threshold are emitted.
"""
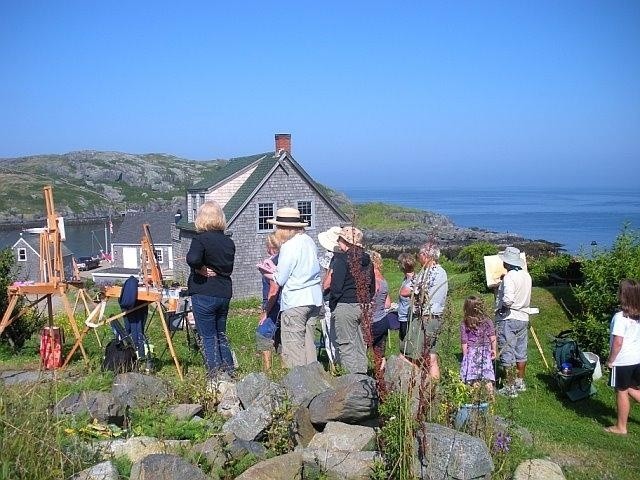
[400,242,448,380]
[256,232,283,371]
[494,247,532,399]
[398,253,418,343]
[365,250,391,374]
[603,278,640,434]
[318,226,375,376]
[265,208,323,370]
[185,200,236,380]
[459,295,497,391]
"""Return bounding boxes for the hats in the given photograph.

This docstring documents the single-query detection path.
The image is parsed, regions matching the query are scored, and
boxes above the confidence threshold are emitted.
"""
[318,226,344,254]
[266,208,309,227]
[498,247,525,267]
[334,227,365,249]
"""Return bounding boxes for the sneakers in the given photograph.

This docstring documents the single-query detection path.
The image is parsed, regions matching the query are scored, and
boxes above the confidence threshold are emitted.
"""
[495,385,518,400]
[145,357,161,374]
[513,378,526,391]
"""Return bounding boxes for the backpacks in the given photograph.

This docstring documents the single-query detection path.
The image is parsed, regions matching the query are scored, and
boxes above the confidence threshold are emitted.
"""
[101,339,136,373]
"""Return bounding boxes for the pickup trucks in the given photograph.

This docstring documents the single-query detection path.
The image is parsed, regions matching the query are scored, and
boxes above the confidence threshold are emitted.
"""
[77,256,99,271]
